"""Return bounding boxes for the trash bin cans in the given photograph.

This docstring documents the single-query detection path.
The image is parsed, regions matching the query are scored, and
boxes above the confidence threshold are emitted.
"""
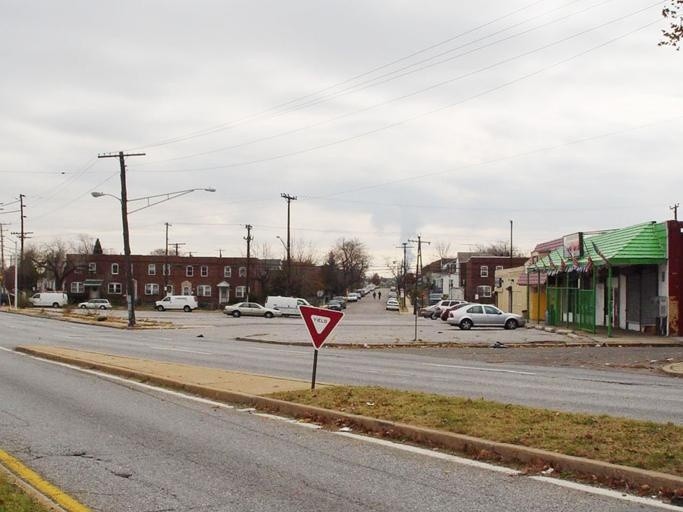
[208,302,217,310]
[521,310,527,319]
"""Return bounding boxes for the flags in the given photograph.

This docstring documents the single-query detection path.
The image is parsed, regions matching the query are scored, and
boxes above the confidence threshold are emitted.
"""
[544,254,593,276]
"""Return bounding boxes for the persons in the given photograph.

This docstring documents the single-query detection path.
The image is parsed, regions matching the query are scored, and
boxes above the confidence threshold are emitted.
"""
[377,291,381,299]
[372,291,376,299]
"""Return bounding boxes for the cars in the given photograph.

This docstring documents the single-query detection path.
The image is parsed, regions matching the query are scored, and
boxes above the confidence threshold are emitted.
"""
[386,285,400,310]
[328,284,380,311]
[77,298,112,309]
[223,301,283,318]
[418,293,525,330]
[0,288,14,306]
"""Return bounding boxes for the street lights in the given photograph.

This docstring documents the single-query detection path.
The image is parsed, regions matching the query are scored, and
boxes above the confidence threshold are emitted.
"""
[91,187,216,214]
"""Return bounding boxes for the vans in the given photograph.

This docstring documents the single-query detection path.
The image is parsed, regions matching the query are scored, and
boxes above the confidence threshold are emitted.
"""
[26,290,69,308]
[155,295,199,312]
[265,295,314,317]
